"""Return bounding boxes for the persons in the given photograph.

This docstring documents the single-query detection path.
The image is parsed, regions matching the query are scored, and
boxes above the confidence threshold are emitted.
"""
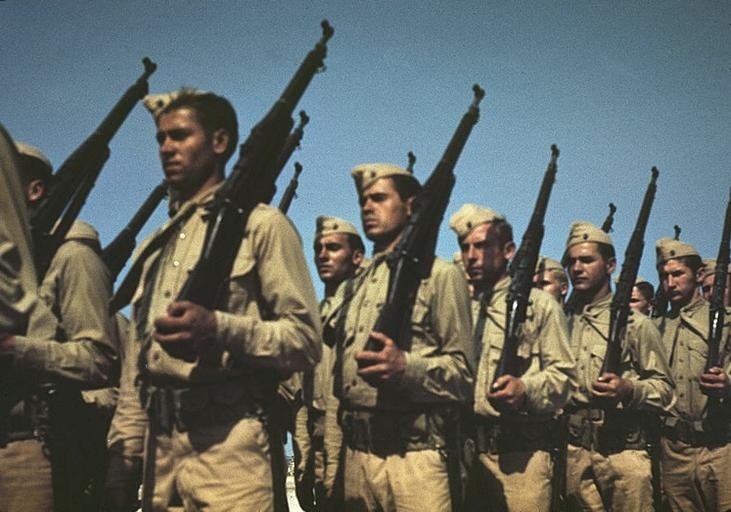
[1,92,731,510]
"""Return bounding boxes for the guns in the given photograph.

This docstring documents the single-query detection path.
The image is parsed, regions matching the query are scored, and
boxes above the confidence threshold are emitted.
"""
[358,85,486,366]
[155,20,335,362]
[104,176,173,273]
[491,145,559,395]
[593,168,659,395]
[25,58,157,282]
[700,199,731,393]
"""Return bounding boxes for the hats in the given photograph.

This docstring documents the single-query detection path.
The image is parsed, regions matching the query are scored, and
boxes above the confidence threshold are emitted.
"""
[566,222,615,249]
[447,202,506,242]
[142,87,209,121]
[702,257,731,277]
[350,163,423,195]
[313,214,368,243]
[654,238,699,269]
[533,255,565,275]
